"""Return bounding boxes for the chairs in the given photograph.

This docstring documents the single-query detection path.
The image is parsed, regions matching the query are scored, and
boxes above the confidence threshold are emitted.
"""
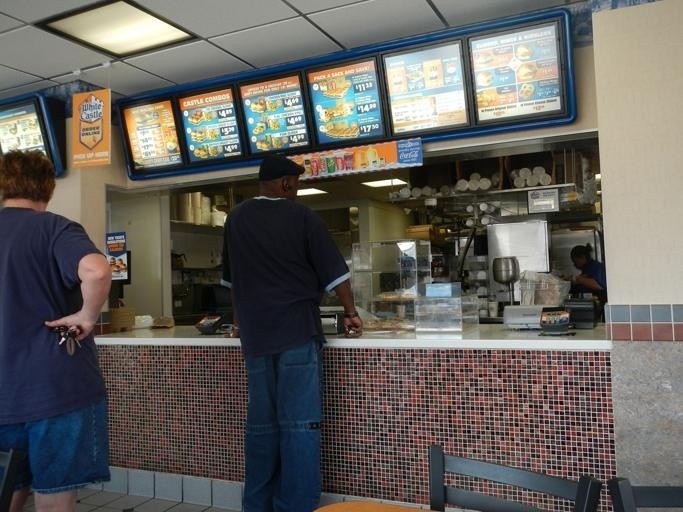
[428,443,683,512]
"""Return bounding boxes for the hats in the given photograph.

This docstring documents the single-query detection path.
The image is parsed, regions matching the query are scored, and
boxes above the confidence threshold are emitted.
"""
[260,156,305,181]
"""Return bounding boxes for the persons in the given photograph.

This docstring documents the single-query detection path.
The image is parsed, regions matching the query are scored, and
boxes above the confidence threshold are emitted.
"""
[0,149,116,511]
[567,243,606,321]
[220,156,359,511]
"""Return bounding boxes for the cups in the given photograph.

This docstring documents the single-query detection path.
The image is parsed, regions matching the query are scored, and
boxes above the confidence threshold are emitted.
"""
[397,305,406,318]
[311,152,354,177]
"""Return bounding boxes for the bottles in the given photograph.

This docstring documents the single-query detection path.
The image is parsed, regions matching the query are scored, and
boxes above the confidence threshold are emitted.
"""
[304,160,312,176]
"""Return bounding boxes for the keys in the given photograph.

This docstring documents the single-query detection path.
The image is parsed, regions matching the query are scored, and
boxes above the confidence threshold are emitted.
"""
[68,332,82,348]
[57,331,69,345]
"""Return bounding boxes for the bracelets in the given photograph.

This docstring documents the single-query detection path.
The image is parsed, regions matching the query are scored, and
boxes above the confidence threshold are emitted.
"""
[342,311,358,318]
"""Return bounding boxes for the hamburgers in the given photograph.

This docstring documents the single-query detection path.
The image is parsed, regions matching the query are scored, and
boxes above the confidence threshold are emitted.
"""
[474,50,493,66]
[191,130,205,143]
[477,72,493,87]
[517,45,533,61]
[517,64,537,81]
[194,149,207,159]
[251,102,265,112]
[255,140,272,152]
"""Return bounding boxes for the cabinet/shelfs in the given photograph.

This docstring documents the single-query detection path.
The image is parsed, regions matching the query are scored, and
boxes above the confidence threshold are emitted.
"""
[407,152,558,197]
